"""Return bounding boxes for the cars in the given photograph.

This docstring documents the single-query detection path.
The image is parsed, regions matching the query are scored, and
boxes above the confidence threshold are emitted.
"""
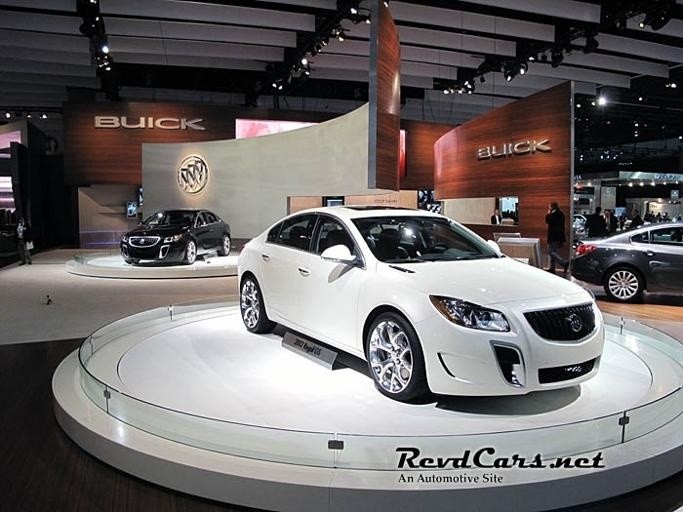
[623,219,651,230]
[115,205,233,266]
[573,213,589,240]
[233,203,608,405]
[567,218,682,304]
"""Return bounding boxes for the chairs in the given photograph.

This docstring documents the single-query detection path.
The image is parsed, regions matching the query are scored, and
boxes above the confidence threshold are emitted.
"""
[289,225,409,259]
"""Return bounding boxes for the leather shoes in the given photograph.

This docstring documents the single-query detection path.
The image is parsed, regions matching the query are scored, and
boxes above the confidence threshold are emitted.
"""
[563,262,569,274]
[546,268,555,274]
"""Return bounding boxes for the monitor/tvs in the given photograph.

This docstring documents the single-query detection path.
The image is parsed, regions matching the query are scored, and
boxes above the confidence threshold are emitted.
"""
[137,187,143,206]
[126,201,138,220]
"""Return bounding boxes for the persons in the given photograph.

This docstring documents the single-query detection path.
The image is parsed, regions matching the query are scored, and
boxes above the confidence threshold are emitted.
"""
[5,213,31,265]
[582,206,670,239]
[490,209,501,224]
[544,202,568,274]
